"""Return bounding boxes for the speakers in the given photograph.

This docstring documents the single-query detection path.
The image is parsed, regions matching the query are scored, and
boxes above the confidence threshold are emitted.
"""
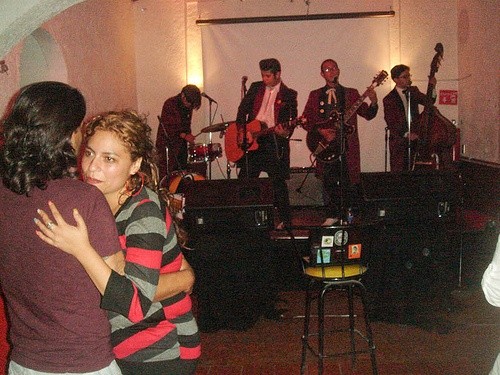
[285,167,324,207]
[349,172,455,225]
[183,177,276,236]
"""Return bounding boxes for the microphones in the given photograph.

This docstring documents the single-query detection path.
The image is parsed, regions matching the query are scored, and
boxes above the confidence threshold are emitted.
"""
[334,76,340,85]
[201,92,217,104]
[408,81,412,88]
[241,76,248,91]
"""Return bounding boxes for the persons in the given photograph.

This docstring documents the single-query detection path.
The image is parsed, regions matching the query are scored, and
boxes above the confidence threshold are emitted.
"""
[382,64,438,185]
[34,109,201,375]
[0,81,196,375]
[301,58,379,227]
[235,57,299,233]
[156,85,201,193]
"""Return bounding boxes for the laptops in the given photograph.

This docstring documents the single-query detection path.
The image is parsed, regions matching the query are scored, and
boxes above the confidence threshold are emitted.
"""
[303,224,368,267]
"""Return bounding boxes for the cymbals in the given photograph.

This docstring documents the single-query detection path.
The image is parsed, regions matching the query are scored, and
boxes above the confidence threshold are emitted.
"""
[202,121,235,132]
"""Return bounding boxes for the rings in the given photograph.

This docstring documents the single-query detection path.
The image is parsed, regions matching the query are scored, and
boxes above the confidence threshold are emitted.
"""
[46,221,54,229]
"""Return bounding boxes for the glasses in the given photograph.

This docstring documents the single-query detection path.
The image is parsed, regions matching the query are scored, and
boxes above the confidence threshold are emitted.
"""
[324,67,337,73]
[397,74,410,78]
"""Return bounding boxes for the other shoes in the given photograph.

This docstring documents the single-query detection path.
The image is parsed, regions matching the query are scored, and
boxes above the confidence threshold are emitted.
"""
[322,217,338,225]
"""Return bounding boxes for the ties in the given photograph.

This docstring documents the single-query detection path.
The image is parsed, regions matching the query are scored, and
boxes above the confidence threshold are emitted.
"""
[402,89,410,102]
[326,88,337,105]
[259,87,273,121]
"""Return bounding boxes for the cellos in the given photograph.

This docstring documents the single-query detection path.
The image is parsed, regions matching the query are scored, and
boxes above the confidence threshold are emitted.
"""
[403,43,457,169]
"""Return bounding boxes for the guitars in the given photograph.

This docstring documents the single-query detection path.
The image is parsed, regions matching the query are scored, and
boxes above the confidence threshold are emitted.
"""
[306,70,389,163]
[225,117,303,163]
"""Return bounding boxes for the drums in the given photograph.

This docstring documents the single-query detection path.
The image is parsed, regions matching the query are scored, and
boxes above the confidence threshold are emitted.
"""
[188,143,222,161]
